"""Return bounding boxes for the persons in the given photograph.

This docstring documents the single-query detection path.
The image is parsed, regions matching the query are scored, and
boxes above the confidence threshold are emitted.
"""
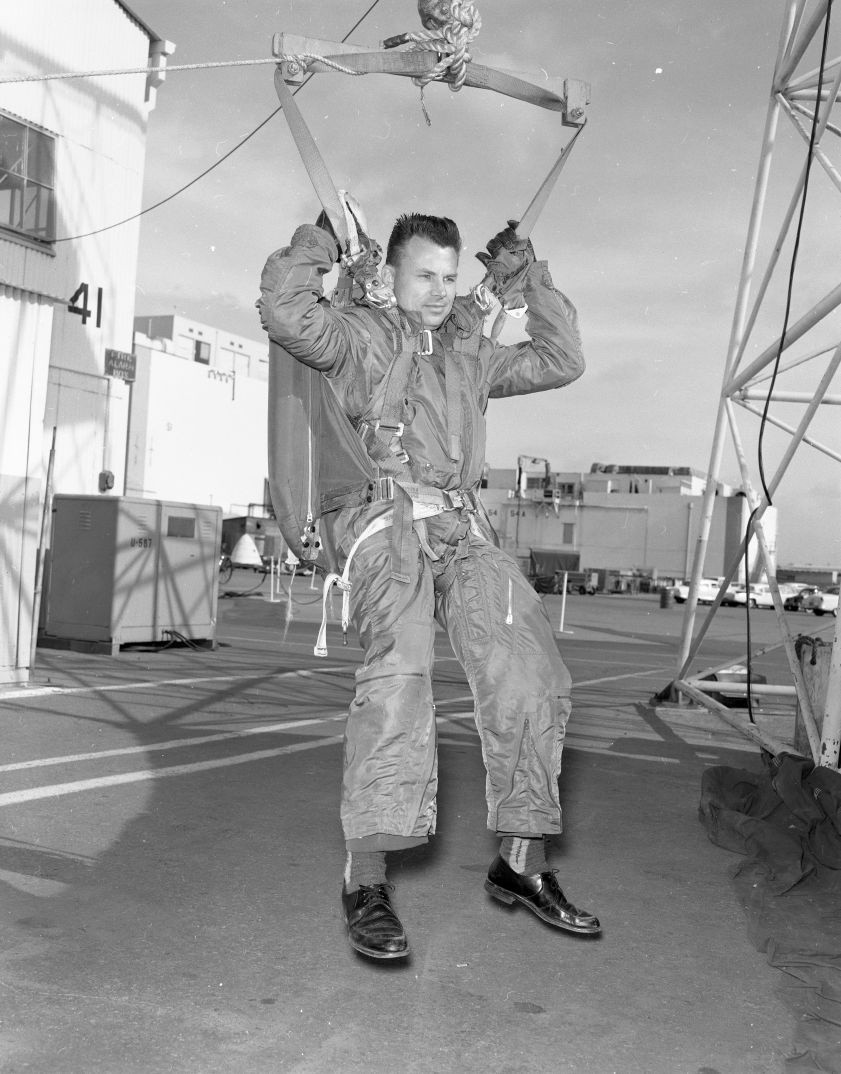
[256,201,601,960]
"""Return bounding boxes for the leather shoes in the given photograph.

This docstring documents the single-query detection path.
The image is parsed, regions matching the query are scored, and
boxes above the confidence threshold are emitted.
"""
[341,877,410,959]
[483,850,602,932]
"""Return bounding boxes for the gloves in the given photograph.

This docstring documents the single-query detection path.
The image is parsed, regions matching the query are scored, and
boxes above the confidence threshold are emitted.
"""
[315,203,362,263]
[475,220,537,278]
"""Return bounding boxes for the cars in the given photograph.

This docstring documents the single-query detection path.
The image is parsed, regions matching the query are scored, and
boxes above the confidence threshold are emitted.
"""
[786,583,809,598]
[672,579,735,606]
[733,583,800,611]
[259,555,281,574]
[800,586,840,617]
[784,587,821,611]
